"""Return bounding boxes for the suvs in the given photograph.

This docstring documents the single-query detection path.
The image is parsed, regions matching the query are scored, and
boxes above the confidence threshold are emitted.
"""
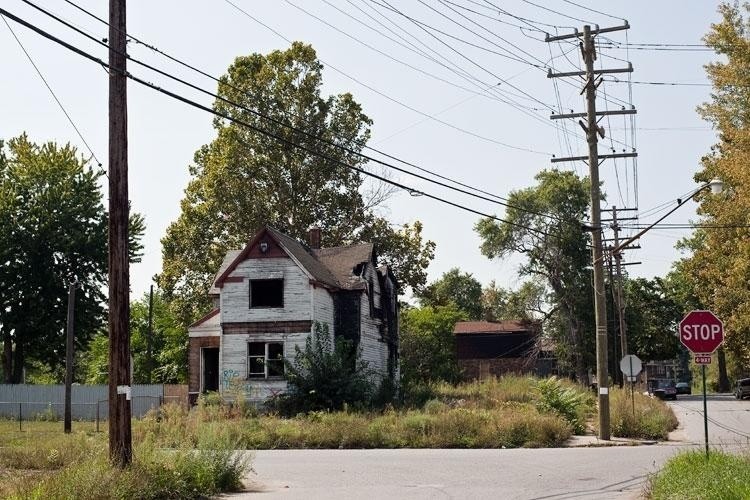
[736,378,750,399]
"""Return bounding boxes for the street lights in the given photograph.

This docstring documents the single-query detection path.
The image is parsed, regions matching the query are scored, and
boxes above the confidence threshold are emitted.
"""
[593,180,723,441]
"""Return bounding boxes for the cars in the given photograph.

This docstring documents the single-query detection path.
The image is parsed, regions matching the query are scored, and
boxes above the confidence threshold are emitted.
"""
[676,382,691,394]
[654,383,677,399]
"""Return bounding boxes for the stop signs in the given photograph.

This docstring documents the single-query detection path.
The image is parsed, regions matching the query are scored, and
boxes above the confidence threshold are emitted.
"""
[679,309,726,356]
